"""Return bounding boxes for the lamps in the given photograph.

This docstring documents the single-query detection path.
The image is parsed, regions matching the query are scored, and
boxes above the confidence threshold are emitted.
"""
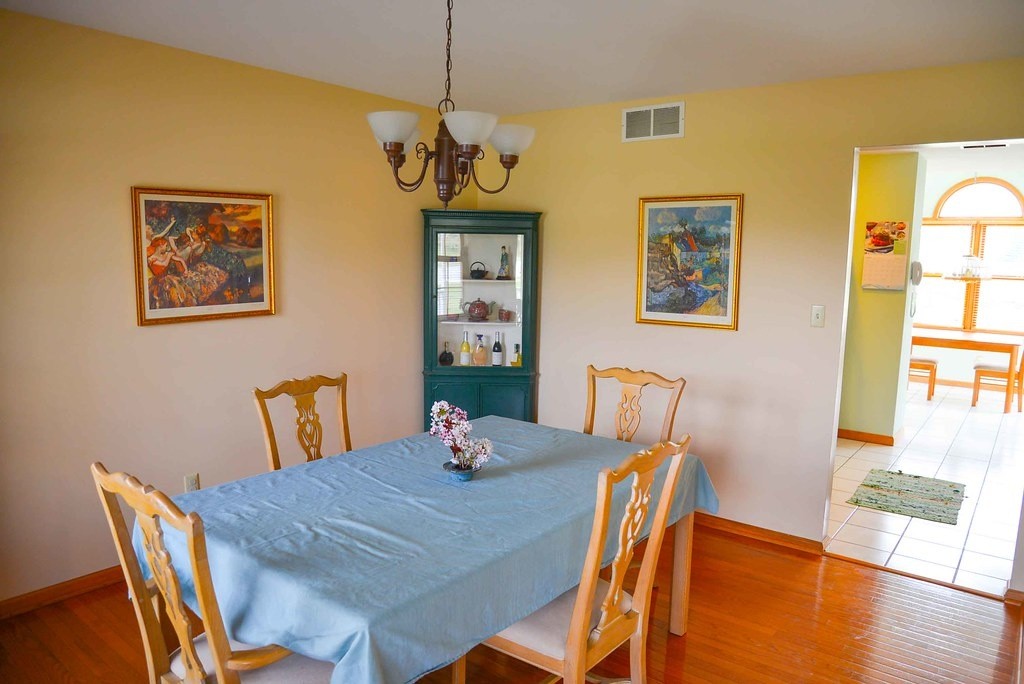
[940,173,992,282]
[366,0,535,212]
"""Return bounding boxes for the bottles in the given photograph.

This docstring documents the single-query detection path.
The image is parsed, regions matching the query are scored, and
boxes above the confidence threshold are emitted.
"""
[510,343,521,366]
[491,332,502,366]
[472,334,487,366]
[439,342,454,366]
[459,331,471,365]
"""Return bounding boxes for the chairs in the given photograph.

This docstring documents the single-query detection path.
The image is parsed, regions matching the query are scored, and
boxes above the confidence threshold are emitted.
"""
[252,373,352,471]
[90,462,337,684]
[583,364,687,587]
[972,350,1024,412]
[910,357,937,400]
[450,432,692,684]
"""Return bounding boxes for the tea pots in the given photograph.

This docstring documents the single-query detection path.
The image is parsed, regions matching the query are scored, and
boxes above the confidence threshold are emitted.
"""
[469,261,489,279]
[462,298,496,322]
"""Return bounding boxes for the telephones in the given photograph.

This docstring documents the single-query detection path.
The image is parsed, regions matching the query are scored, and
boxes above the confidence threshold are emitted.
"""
[911,260,922,286]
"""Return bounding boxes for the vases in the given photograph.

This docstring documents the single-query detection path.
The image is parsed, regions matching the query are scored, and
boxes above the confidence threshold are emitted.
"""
[442,462,482,482]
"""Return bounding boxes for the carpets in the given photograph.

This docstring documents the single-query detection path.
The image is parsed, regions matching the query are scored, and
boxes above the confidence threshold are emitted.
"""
[845,467,965,525]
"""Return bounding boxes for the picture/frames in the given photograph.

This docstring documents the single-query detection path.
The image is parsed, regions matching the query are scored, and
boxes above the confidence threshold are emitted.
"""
[130,185,275,326]
[636,194,745,332]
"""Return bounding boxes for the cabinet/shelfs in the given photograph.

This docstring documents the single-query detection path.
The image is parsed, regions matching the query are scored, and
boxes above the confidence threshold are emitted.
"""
[419,207,542,433]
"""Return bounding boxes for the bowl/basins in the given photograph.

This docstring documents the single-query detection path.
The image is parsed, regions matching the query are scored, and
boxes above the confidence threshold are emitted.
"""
[447,314,459,322]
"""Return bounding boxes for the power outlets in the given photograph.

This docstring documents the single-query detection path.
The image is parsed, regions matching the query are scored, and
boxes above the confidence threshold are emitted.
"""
[184,472,200,492]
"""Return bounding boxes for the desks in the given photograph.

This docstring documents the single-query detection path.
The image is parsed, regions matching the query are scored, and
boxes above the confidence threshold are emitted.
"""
[912,327,1023,413]
[129,415,719,684]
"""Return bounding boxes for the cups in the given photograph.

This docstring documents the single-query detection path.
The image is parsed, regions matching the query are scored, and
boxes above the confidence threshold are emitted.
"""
[498,309,509,321]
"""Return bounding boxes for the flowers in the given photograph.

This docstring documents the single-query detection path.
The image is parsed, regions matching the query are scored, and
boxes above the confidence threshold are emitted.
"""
[429,399,493,469]
